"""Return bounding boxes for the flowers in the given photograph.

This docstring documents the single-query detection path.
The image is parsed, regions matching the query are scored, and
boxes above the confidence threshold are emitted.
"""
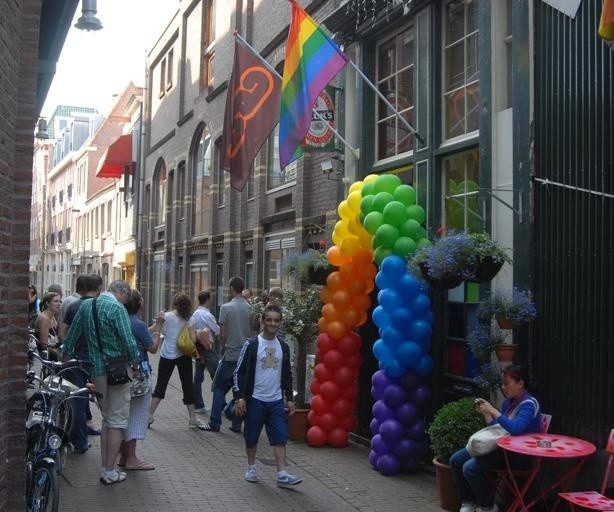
[447,232,514,281]
[403,235,471,295]
[275,248,336,287]
[472,364,504,392]
[466,324,508,356]
[475,287,540,330]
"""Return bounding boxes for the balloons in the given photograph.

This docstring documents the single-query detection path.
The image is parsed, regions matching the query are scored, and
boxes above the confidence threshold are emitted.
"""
[308,174,435,477]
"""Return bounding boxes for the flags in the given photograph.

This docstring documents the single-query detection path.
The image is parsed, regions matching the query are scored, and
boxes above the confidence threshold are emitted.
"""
[219,39,281,192]
[278,1,351,170]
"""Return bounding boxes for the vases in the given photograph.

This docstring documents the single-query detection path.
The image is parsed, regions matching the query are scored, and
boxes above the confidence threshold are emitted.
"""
[461,257,504,283]
[417,260,460,289]
[494,342,515,361]
[497,316,515,332]
[306,268,328,287]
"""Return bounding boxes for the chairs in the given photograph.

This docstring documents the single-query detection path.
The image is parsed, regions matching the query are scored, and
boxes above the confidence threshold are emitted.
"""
[484,412,553,511]
[556,426,614,511]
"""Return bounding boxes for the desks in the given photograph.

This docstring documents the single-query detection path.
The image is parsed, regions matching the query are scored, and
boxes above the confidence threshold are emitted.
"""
[496,432,596,512]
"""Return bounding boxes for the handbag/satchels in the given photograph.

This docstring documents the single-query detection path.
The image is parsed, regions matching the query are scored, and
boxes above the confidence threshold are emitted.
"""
[466,424,513,457]
[223,398,250,422]
[102,356,129,385]
[128,360,150,398]
[176,322,197,357]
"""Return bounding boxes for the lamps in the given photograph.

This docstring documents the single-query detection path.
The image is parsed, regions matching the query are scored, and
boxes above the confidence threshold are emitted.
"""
[319,159,352,186]
[34,115,48,143]
[72,1,104,32]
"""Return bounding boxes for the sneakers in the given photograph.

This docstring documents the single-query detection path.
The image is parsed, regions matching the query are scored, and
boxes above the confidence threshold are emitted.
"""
[189,406,220,432]
[276,475,303,485]
[245,466,259,482]
[100,469,128,484]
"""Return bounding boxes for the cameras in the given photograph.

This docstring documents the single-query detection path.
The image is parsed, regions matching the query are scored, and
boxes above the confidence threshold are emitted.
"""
[475,398,484,406]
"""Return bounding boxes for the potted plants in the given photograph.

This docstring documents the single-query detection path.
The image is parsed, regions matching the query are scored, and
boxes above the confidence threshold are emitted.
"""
[424,396,495,510]
[274,290,323,441]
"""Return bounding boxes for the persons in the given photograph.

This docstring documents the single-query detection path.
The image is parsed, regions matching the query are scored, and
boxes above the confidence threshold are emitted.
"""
[449,364,542,511]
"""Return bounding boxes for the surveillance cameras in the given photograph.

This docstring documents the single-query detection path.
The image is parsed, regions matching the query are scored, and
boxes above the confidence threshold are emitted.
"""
[321,160,333,174]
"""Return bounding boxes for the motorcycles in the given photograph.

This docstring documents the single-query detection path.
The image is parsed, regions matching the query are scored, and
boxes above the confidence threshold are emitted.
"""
[26,330,103,512]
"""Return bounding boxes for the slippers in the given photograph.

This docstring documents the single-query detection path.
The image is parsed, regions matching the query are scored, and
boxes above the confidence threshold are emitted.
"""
[125,461,155,470]
